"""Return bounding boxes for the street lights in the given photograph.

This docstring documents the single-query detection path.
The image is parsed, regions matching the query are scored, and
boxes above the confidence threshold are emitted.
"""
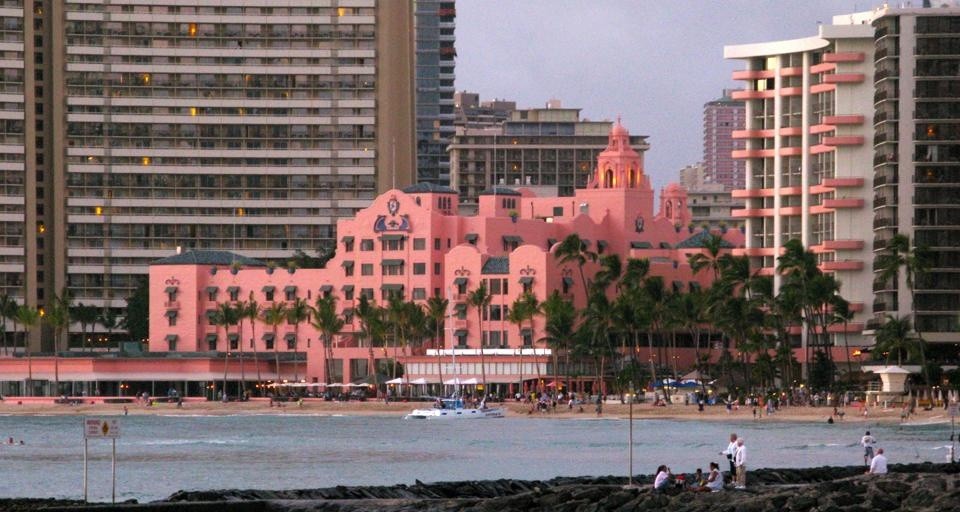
[673,354,680,380]
[852,350,861,361]
[648,354,656,362]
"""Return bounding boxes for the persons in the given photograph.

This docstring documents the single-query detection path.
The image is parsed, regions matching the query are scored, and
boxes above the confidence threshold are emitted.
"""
[900,398,917,417]
[863,448,889,474]
[682,462,724,493]
[515,389,607,417]
[753,409,756,417]
[832,407,844,417]
[858,432,877,467]
[723,390,844,407]
[654,465,675,489]
[733,437,746,489]
[718,433,738,485]
[863,407,868,415]
[434,390,505,409]
[694,467,701,484]
[654,392,666,407]
[943,396,948,410]
[685,394,689,406]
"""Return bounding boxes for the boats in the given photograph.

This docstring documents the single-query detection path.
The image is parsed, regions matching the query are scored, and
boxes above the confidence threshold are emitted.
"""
[405,398,504,420]
